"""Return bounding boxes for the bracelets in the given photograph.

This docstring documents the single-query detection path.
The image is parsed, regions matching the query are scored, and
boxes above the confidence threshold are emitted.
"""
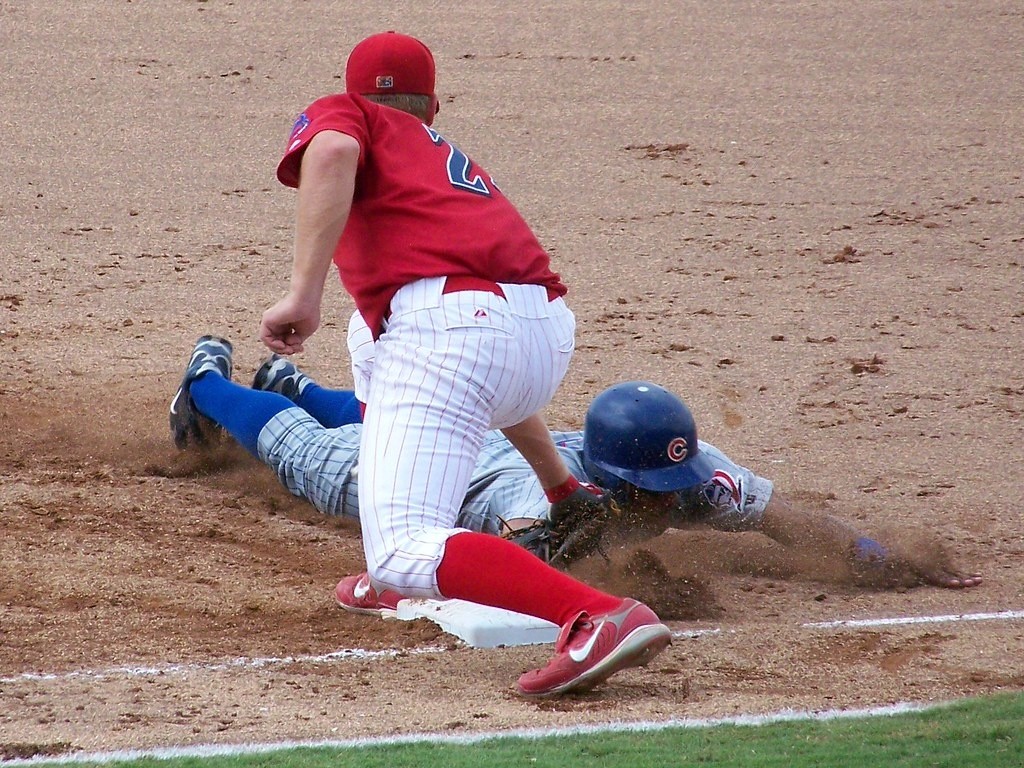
[545,474,578,503]
[859,539,887,565]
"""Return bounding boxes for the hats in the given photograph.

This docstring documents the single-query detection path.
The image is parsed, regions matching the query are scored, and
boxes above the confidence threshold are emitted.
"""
[346,30,439,115]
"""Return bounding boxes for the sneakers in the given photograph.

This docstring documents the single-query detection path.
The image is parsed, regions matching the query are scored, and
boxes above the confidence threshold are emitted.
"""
[514,598,672,701]
[169,335,233,453]
[251,351,314,407]
[335,572,409,619]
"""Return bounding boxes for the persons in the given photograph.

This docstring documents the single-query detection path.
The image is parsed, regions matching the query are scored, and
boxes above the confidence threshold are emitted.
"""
[169,334,984,589]
[258,31,671,699]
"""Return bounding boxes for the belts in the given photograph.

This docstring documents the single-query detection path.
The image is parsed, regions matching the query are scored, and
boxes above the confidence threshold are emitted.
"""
[384,275,561,320]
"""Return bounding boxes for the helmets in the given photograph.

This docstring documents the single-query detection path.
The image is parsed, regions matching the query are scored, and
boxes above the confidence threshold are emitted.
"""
[582,381,715,492]
